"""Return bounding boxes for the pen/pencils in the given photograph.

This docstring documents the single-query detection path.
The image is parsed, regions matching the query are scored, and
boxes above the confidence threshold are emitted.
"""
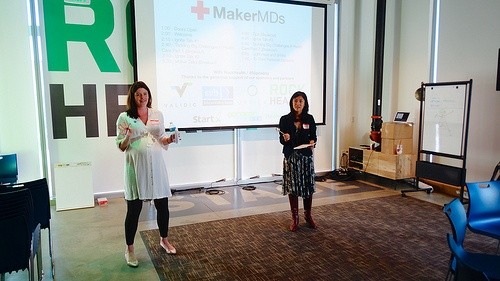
[276,128,284,135]
[125,123,131,135]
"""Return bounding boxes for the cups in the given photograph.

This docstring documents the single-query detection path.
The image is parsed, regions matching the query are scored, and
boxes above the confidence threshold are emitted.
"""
[396,144,403,155]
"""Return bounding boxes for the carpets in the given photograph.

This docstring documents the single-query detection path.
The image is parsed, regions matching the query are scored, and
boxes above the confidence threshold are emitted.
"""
[139,197,500,281]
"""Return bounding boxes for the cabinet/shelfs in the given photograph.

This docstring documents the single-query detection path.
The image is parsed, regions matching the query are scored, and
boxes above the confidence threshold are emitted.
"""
[348,147,378,175]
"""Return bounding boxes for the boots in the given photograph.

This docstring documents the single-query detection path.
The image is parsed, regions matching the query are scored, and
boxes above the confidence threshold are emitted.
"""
[290,208,299,231]
[303,210,317,227]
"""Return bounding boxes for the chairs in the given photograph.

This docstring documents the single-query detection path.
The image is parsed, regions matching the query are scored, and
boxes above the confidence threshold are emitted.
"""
[465,180,500,254]
[442,198,483,281]
[445,233,500,281]
[0,154,53,281]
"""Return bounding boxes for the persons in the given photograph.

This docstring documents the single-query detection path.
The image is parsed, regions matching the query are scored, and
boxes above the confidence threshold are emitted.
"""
[117,81,181,267]
[277,91,318,230]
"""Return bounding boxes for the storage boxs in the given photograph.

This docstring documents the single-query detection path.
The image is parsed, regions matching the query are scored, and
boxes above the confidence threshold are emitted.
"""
[378,154,416,180]
[381,139,414,154]
[381,122,413,140]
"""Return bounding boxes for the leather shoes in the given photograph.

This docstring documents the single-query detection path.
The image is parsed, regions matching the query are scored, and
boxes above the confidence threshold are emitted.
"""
[160,240,176,254]
[125,251,139,267]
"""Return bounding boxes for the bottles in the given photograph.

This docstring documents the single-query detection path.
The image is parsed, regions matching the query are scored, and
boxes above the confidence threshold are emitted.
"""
[169,122,177,142]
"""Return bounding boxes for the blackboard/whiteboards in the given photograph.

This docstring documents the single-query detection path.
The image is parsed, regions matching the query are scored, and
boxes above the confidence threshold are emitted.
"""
[418,79,473,160]
